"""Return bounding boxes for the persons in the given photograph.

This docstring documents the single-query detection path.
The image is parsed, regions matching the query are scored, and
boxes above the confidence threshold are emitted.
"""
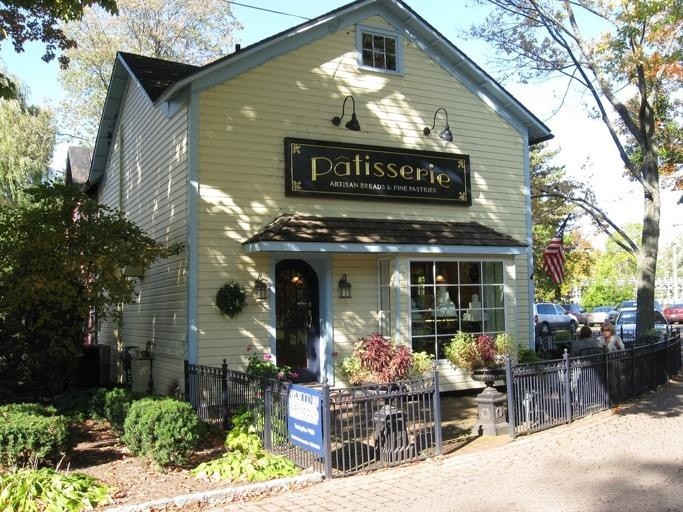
[570,326,602,361]
[596,322,625,360]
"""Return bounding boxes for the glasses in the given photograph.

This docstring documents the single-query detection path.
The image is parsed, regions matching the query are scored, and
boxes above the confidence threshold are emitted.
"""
[601,328,610,332]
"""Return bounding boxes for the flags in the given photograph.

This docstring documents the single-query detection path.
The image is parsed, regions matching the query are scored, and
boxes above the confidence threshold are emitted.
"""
[543,217,570,284]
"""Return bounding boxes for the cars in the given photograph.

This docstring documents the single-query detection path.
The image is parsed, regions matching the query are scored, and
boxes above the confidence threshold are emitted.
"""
[534,302,578,336]
[561,303,616,327]
[613,299,682,343]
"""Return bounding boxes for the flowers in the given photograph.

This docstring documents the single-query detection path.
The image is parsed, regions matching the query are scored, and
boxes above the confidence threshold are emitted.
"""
[244,344,299,389]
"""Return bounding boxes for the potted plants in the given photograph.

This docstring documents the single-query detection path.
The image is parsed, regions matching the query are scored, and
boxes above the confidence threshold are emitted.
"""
[339,330,514,464]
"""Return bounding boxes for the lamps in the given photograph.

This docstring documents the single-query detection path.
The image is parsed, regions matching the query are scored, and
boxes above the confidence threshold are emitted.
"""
[254,273,267,300]
[331,94,360,132]
[338,273,351,299]
[422,107,453,143]
